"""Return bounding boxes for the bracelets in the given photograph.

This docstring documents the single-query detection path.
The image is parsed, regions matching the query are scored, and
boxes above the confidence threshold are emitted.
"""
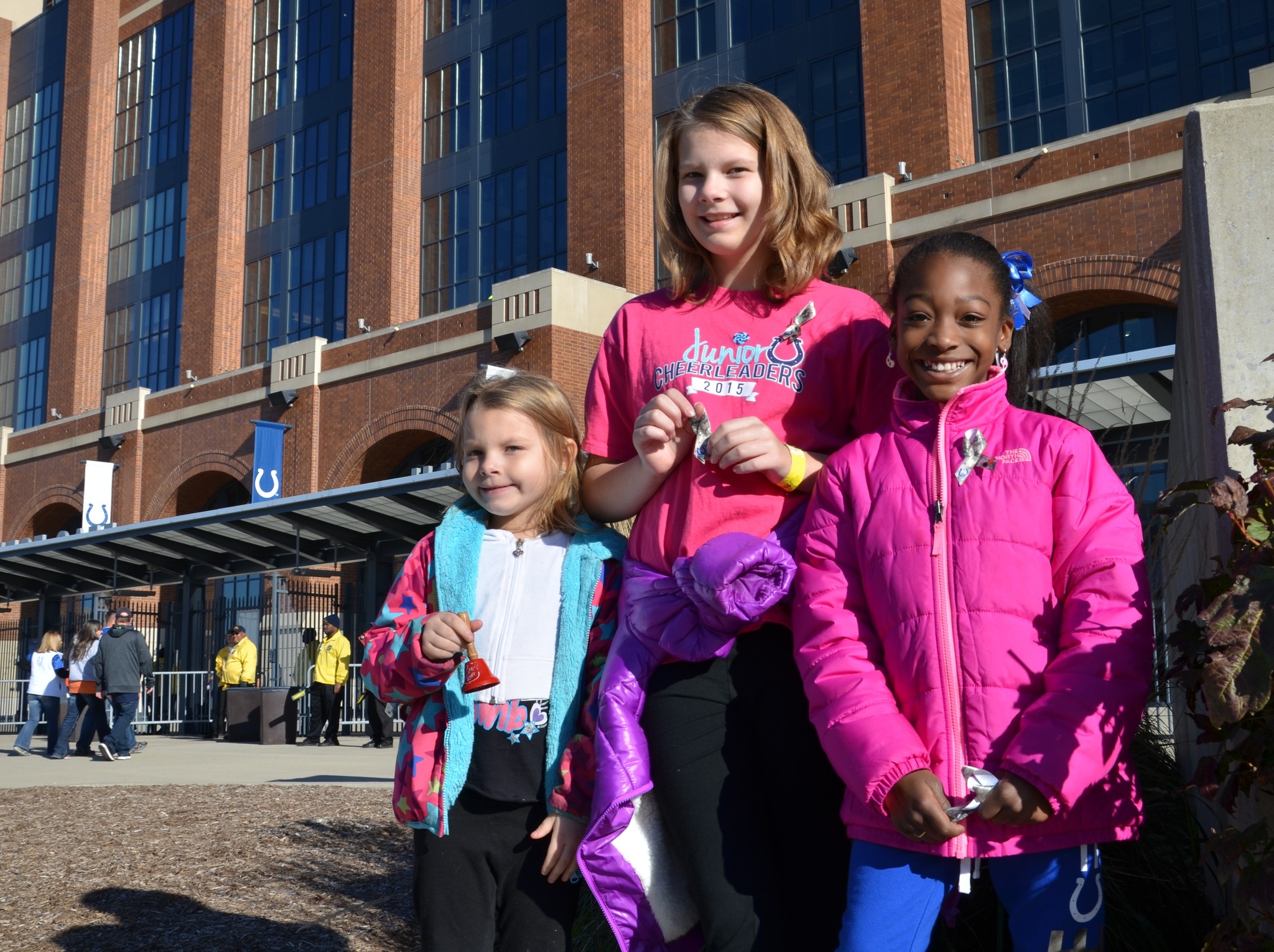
[770,441,806,496]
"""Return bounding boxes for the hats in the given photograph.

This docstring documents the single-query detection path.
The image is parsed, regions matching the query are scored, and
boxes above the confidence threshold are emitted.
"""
[323,613,340,629]
[230,625,245,633]
[116,606,132,618]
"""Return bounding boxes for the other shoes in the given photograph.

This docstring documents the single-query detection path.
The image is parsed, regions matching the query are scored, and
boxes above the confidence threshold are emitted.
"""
[205,731,218,738]
[223,735,228,740]
[50,753,71,759]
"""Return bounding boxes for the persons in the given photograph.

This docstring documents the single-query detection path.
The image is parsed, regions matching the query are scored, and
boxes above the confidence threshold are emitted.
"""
[52,619,132,761]
[357,364,637,952]
[96,610,148,755]
[149,648,180,734]
[203,629,240,740]
[97,608,156,762]
[12,631,72,759]
[582,67,902,952]
[789,226,1156,951]
[295,612,352,747]
[220,624,256,688]
[362,687,395,749]
[292,628,321,736]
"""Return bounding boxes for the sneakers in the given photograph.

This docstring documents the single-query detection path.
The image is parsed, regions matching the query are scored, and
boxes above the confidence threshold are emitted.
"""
[117,753,131,760]
[13,746,31,756]
[98,740,115,761]
[74,746,95,756]
[129,741,147,753]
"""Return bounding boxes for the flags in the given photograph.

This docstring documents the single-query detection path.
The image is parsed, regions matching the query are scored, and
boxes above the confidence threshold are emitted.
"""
[80,460,115,530]
[251,420,286,505]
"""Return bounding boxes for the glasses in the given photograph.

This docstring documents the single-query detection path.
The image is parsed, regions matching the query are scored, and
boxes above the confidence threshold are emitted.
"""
[232,633,242,635]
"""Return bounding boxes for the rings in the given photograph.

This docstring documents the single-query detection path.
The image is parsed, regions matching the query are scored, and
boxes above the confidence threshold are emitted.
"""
[914,832,926,838]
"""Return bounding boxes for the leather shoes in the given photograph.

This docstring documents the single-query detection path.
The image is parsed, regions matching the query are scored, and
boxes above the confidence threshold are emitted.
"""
[298,738,320,746]
[378,740,393,748]
[318,738,340,747]
[362,739,380,748]
